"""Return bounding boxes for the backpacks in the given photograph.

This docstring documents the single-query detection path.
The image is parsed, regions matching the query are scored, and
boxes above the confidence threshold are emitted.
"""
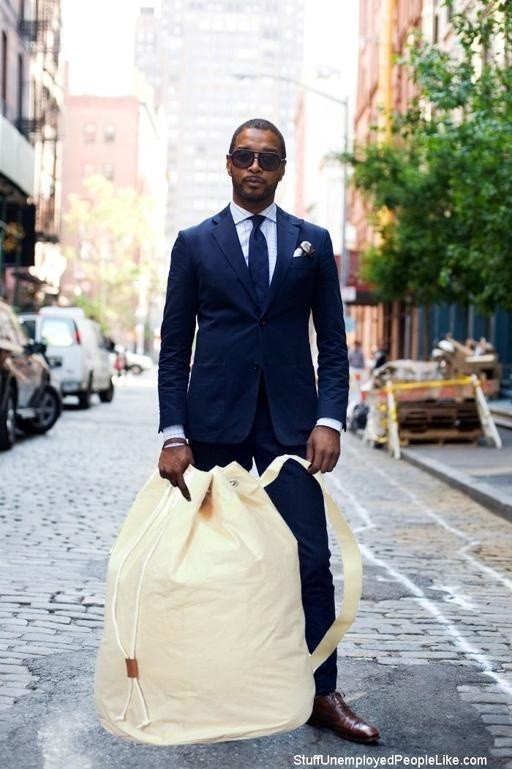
[94,454,363,749]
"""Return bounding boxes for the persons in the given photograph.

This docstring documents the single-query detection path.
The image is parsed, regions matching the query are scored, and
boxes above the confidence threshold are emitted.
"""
[371,348,386,369]
[156,118,380,743]
[349,339,364,368]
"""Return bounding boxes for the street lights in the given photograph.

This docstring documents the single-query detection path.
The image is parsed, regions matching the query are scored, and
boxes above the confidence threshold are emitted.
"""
[229,67,350,323]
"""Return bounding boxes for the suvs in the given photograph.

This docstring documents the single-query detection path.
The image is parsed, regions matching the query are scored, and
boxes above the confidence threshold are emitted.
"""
[16,304,117,409]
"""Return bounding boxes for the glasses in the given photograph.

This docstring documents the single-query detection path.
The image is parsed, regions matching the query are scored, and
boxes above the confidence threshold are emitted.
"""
[226,150,286,172]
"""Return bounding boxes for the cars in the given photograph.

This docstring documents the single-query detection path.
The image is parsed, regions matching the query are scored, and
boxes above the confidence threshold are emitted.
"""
[109,344,154,376]
[0,298,63,452]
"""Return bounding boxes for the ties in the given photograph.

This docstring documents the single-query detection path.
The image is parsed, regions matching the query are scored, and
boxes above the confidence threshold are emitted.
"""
[248,214,270,319]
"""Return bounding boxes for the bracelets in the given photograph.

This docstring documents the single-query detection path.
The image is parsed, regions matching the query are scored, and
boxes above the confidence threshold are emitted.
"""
[162,436,188,447]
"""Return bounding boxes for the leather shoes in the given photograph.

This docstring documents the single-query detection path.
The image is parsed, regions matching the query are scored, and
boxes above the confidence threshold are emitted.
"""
[307,692,381,745]
